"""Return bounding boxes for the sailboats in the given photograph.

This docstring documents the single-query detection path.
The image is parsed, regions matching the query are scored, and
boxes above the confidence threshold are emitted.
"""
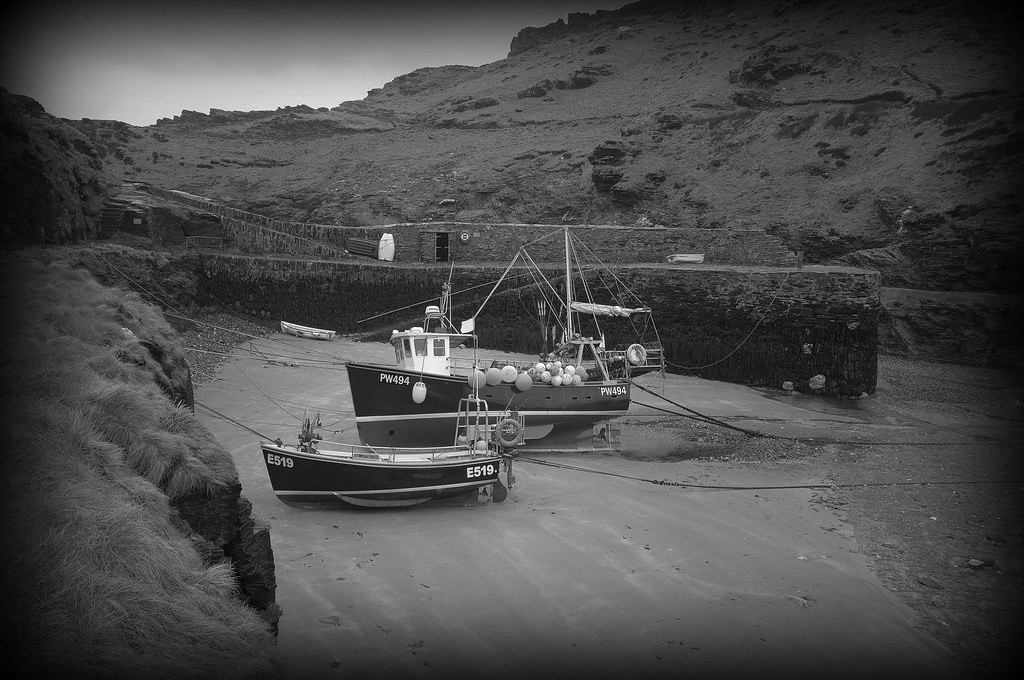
[345,224,667,455]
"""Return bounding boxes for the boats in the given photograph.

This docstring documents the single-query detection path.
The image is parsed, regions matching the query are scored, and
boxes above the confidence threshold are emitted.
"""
[665,253,706,264]
[259,329,526,513]
[280,319,336,341]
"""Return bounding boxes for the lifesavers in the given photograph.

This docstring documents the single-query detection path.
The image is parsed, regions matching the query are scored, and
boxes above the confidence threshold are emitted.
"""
[626,343,646,366]
[496,419,523,447]
